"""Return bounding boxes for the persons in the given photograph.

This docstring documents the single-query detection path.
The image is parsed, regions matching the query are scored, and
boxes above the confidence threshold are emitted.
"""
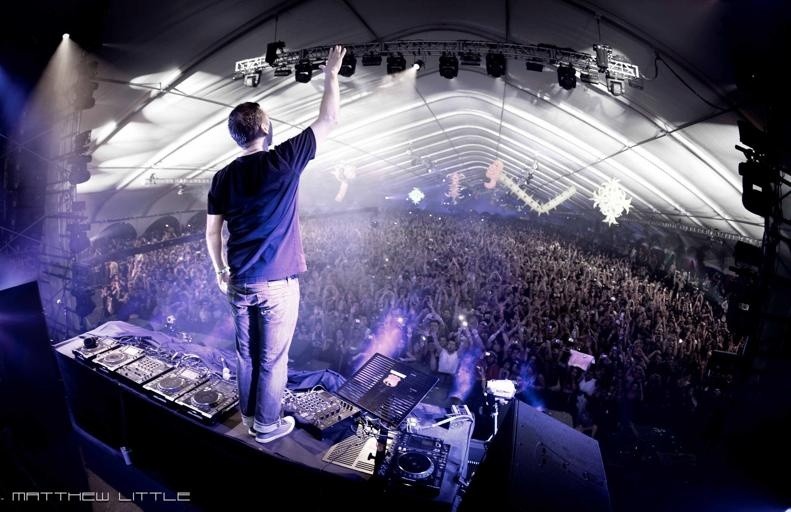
[88,207,742,439]
[203,44,347,444]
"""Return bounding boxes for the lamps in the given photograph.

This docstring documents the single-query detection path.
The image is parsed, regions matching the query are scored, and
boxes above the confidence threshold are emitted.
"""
[412,59,424,71]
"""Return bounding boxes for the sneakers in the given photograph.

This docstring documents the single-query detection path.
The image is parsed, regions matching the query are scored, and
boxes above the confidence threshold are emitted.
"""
[255,415,296,444]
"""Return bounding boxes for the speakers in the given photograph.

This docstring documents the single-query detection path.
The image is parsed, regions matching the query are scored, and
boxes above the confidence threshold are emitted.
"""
[455,399,612,512]
